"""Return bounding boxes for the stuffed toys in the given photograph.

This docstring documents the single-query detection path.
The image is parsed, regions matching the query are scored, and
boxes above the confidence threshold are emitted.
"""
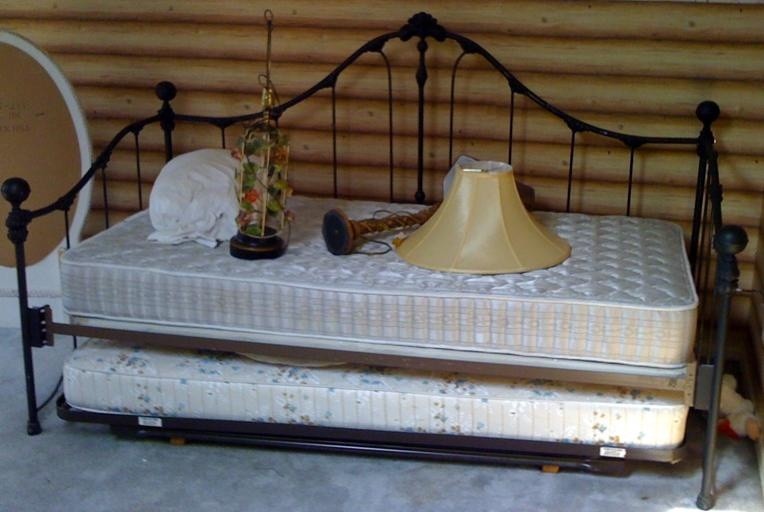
[716,373,762,443]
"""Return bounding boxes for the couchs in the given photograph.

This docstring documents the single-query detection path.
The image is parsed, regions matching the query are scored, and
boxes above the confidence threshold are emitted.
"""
[6,12,745,511]
